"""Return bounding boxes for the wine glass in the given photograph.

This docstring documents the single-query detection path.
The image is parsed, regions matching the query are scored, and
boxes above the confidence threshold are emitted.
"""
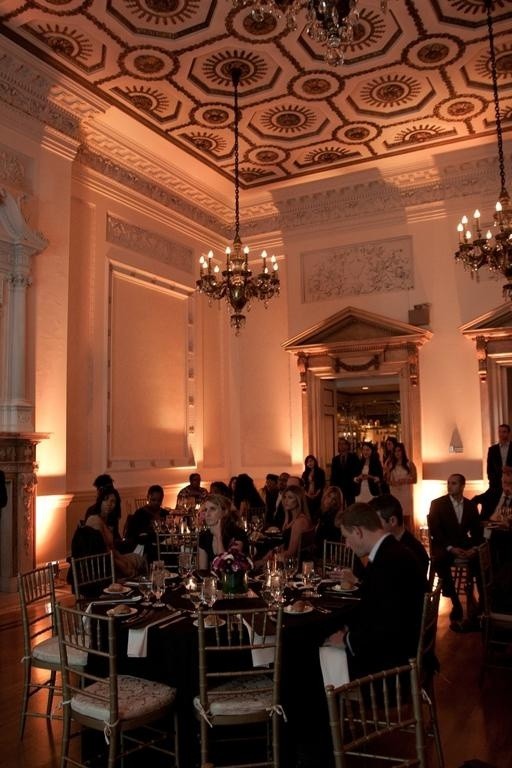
[136,552,322,618]
[153,502,282,542]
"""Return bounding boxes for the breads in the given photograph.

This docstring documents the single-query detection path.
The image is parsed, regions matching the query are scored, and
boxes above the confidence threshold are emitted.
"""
[107,583,123,592]
[115,605,130,614]
[290,600,304,612]
[205,615,219,625]
[340,581,353,590]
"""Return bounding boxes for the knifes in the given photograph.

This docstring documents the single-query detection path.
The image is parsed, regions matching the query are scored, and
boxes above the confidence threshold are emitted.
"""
[331,596,360,601]
[159,616,187,629]
[93,603,137,606]
[269,615,285,628]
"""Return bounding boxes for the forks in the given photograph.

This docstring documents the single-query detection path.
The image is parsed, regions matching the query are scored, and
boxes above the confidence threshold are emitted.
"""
[120,608,149,624]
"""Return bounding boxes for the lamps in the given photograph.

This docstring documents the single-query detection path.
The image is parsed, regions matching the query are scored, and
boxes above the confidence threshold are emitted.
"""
[231,0,360,69]
[196,68,280,341]
[454,0,512,303]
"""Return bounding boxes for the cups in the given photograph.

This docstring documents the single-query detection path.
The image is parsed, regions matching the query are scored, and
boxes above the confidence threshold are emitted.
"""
[496,507,512,527]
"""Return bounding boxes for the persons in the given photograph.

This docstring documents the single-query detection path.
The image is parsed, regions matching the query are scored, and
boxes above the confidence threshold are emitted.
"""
[70,425,512,718]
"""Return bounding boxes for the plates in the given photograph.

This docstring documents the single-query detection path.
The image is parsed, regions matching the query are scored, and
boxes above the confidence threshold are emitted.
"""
[102,587,132,594]
[193,618,226,629]
[322,576,358,593]
[106,607,138,617]
